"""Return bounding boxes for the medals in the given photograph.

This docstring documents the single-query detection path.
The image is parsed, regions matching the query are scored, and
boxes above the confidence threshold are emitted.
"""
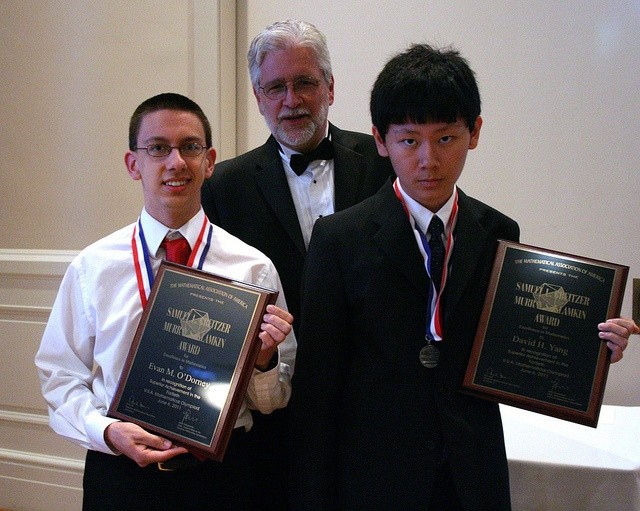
[394,179,458,368]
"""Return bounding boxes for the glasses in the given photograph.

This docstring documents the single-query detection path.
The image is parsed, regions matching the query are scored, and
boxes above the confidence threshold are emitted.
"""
[134,142,207,157]
[258,75,326,100]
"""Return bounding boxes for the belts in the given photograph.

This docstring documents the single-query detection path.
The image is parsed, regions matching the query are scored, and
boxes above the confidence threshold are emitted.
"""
[157,460,179,472]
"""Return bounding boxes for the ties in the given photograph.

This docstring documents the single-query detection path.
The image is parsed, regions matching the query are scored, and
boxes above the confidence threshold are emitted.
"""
[427,214,446,295]
[161,235,192,265]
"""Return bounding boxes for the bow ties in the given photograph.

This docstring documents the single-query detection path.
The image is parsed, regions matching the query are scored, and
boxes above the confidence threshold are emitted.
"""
[275,121,335,177]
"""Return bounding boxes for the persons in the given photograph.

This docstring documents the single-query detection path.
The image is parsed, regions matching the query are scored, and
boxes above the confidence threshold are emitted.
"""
[33,92,296,510]
[201,19,397,510]
[294,44,639,511]
